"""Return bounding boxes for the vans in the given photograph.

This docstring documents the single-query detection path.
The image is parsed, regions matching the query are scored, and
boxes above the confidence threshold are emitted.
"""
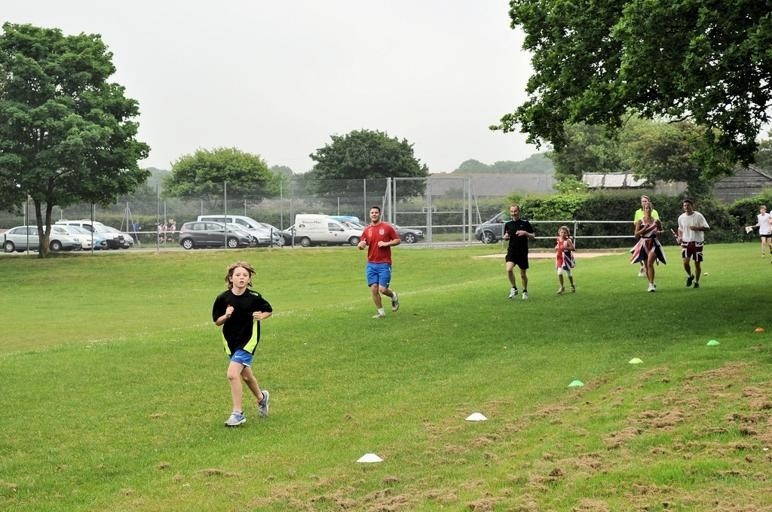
[475,208,508,244]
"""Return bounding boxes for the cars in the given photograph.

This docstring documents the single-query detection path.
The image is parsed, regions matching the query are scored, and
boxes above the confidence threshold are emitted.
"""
[0,219,134,251]
[180,212,424,249]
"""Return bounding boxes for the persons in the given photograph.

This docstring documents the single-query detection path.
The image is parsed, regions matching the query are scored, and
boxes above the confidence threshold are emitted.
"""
[632,195,660,277]
[677,199,711,288]
[132,220,141,244]
[629,201,667,293]
[502,204,535,301]
[212,260,273,426]
[756,205,772,259]
[356,206,402,320]
[159,216,176,243]
[554,226,576,295]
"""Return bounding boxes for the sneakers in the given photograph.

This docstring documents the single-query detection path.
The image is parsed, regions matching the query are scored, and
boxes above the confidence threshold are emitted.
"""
[507,287,520,299]
[685,274,700,288]
[390,291,400,312]
[647,281,656,292]
[761,250,772,264]
[556,286,566,294]
[223,408,248,427]
[638,267,646,277]
[569,283,576,293]
[521,289,528,301]
[257,390,270,418]
[371,311,387,321]
